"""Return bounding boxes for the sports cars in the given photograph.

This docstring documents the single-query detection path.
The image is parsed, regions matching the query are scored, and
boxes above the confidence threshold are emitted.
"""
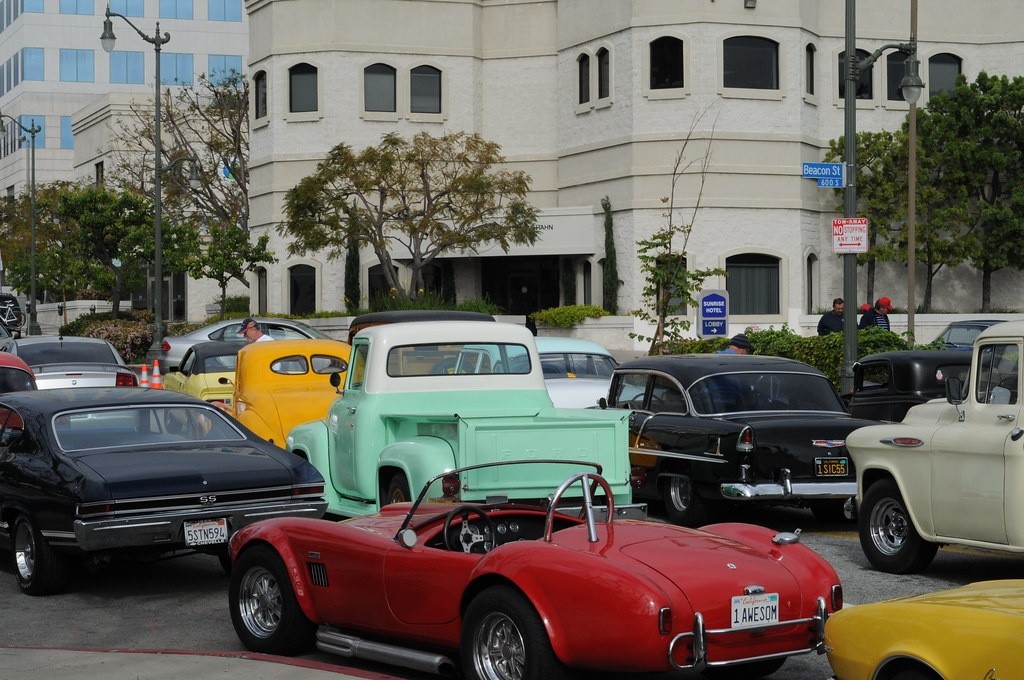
[226,459,842,680]
[824,579,1024,680]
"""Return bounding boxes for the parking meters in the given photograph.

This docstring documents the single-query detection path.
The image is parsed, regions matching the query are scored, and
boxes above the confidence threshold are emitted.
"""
[57,303,63,328]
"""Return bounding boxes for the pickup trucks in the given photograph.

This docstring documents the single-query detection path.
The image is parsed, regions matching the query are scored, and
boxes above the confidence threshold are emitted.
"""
[283,322,632,505]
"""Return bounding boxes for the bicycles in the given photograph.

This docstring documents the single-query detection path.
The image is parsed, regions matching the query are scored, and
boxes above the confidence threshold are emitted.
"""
[1,300,26,330]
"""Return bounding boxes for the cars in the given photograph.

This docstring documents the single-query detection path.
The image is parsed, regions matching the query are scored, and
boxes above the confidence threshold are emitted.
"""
[163,317,331,374]
[162,341,253,404]
[218,339,364,449]
[0,322,326,597]
[348,311,1024,575]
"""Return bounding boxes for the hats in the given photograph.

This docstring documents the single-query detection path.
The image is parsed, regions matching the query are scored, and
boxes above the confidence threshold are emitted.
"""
[236,318,258,334]
[859,303,871,313]
[730,333,752,349]
[879,296,893,310]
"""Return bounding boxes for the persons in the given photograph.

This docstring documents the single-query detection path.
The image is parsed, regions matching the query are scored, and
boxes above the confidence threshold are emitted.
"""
[858,297,893,331]
[714,334,752,355]
[817,298,859,336]
[237,318,281,412]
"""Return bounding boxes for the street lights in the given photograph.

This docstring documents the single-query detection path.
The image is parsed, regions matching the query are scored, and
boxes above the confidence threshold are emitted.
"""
[102,2,201,360]
[0,111,42,335]
[837,1,927,395]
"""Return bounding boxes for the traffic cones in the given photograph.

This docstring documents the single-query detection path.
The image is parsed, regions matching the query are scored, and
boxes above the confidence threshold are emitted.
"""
[151,360,161,389]
[140,364,151,388]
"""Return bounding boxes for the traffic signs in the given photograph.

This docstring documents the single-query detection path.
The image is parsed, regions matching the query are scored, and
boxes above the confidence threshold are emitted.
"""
[701,320,725,336]
[832,218,870,255]
